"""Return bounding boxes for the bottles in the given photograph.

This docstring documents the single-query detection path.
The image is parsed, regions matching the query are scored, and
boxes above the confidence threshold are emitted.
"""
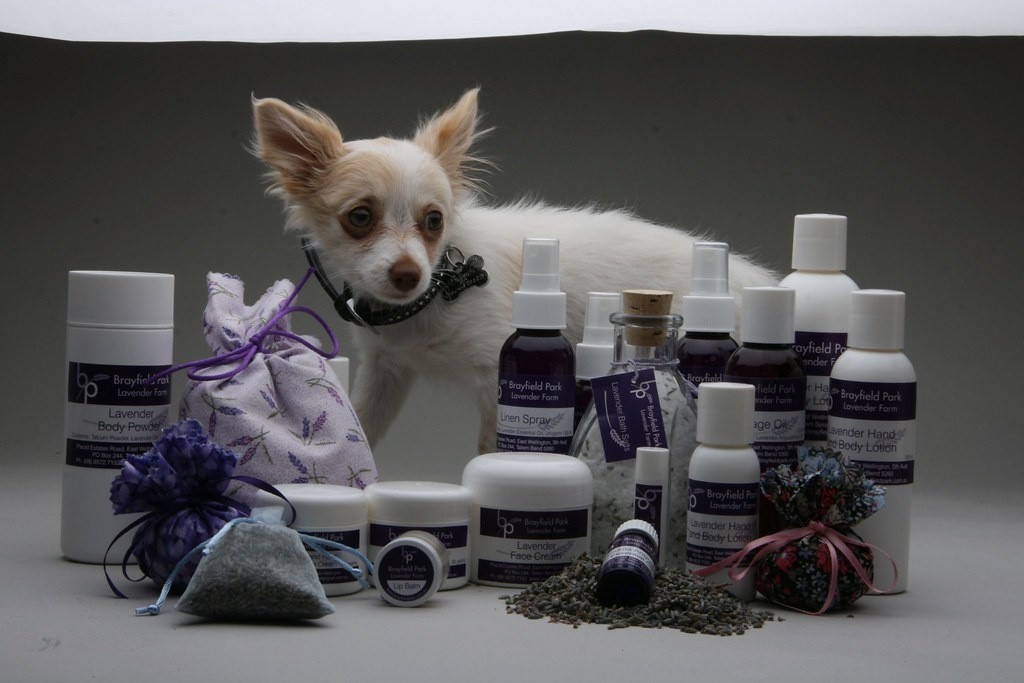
[60,269,175,566]
[495,239,577,456]
[722,286,807,478]
[829,288,918,595]
[597,520,661,607]
[675,241,740,384]
[566,290,697,570]
[778,214,863,452]
[687,382,760,602]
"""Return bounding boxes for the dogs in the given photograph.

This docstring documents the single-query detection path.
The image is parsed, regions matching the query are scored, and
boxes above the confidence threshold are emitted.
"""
[245,84,786,454]
